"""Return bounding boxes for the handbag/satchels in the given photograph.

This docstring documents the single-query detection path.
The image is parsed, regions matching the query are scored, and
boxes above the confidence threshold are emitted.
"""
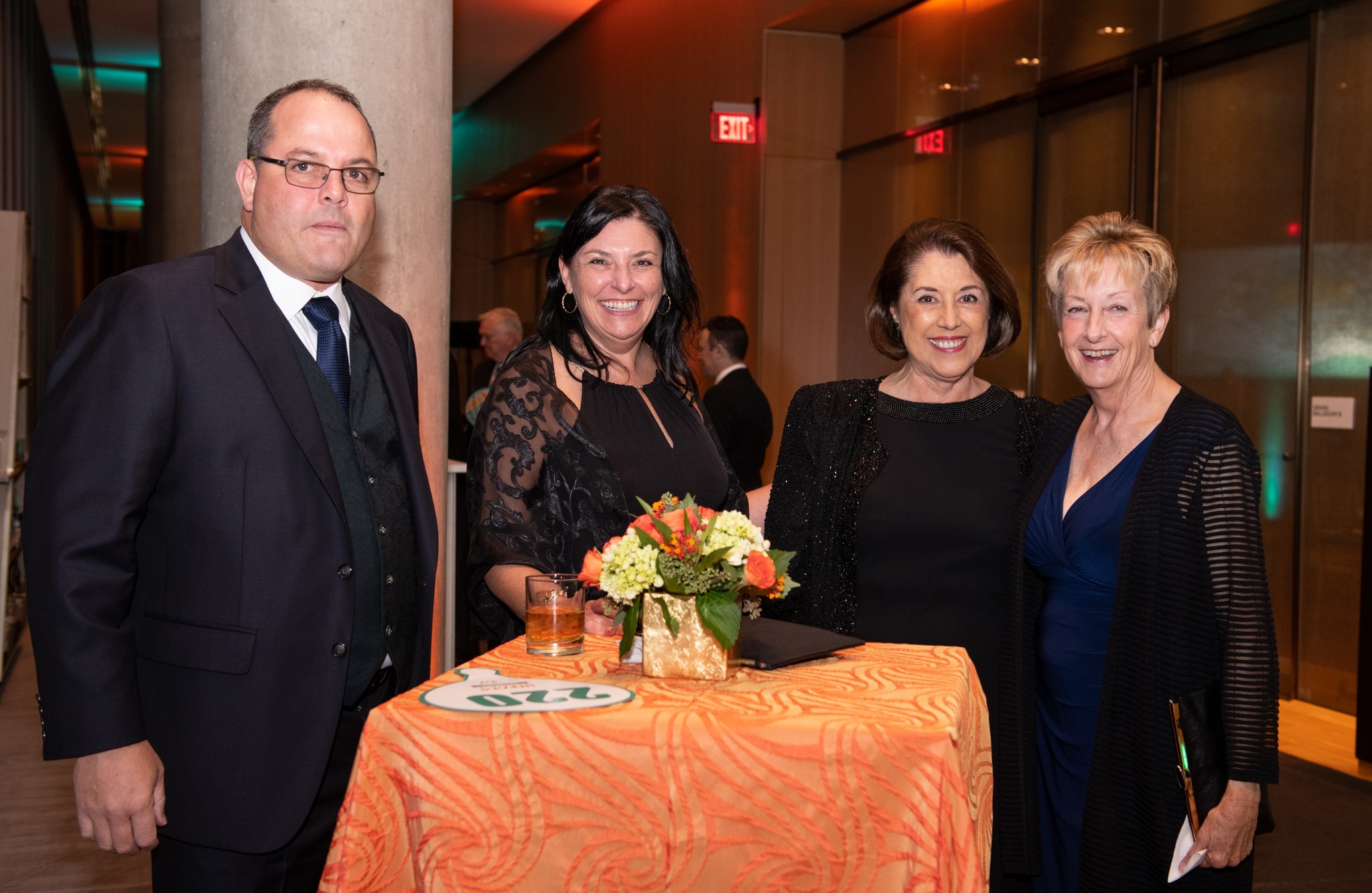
[1168,685,1276,846]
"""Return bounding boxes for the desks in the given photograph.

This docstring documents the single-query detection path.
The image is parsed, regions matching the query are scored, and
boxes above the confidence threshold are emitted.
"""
[317,631,992,893]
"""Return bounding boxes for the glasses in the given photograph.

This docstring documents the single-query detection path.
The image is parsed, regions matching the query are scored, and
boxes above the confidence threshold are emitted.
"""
[249,156,385,194]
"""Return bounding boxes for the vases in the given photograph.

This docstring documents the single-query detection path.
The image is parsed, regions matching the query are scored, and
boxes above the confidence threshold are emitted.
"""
[641,593,742,682]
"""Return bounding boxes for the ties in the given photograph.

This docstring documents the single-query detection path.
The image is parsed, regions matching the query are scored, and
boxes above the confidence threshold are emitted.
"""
[300,295,351,417]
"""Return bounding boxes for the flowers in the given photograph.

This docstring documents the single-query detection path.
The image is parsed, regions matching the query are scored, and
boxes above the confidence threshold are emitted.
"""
[577,499,800,665]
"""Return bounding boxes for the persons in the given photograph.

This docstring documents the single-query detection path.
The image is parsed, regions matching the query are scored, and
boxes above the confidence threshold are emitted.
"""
[760,214,1068,889]
[29,76,439,893]
[460,180,773,665]
[698,313,774,490]
[1021,206,1282,893]
[477,305,526,386]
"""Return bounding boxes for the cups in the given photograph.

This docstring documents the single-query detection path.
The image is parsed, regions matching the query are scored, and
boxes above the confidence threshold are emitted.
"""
[522,572,588,657]
